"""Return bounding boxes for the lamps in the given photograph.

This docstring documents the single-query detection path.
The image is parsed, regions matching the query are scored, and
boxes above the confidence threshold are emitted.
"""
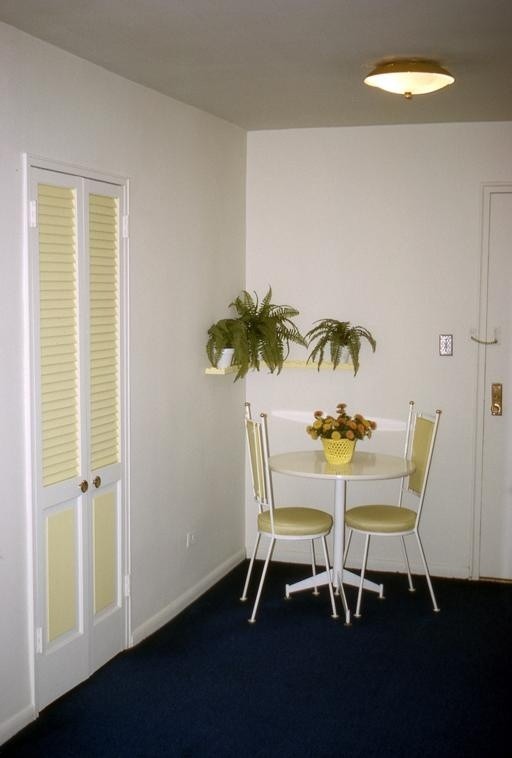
[362,59,454,99]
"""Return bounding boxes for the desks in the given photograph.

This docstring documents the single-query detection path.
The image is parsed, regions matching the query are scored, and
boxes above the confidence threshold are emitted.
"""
[265,451,416,601]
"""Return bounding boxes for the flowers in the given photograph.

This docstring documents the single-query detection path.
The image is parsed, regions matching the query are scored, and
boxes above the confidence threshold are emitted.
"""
[306,402,376,441]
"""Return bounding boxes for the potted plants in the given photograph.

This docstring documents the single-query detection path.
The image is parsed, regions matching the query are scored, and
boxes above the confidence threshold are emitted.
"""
[201,285,377,380]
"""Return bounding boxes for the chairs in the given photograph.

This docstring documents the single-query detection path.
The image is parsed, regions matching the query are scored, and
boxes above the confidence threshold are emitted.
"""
[343,401,439,622]
[243,403,338,622]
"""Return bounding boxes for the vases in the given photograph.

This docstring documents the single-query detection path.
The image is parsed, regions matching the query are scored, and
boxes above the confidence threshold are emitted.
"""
[320,437,356,464]
